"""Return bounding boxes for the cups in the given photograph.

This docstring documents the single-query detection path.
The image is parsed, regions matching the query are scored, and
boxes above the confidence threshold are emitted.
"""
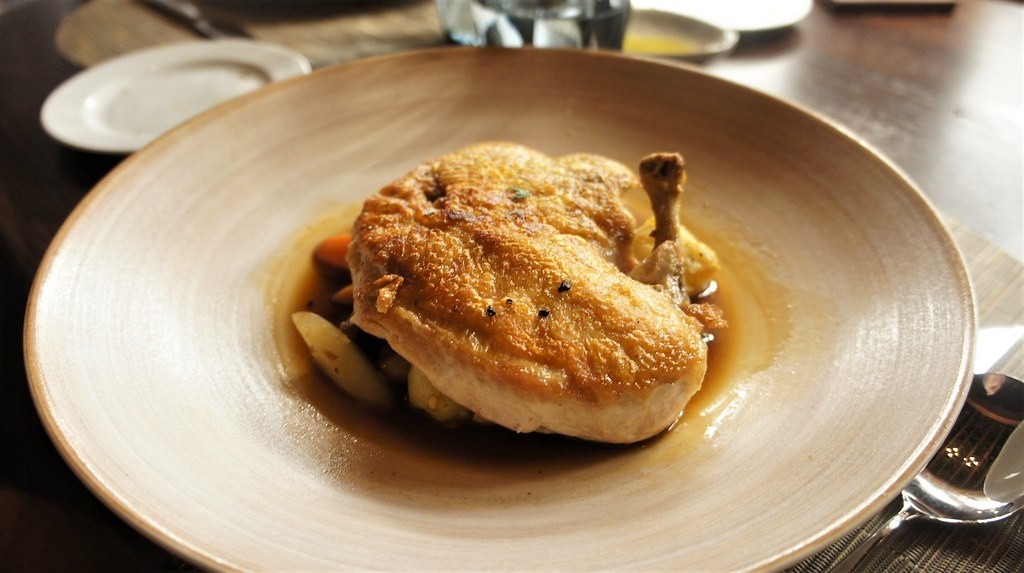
[435,0,631,53]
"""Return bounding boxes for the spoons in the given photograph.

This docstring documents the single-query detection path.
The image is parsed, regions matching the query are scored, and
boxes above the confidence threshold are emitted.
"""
[827,372,1024,573]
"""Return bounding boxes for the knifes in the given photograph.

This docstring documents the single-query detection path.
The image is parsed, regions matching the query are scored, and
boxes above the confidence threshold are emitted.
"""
[143,0,226,40]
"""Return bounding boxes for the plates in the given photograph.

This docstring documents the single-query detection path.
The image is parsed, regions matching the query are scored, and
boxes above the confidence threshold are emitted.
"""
[620,7,739,65]
[632,0,813,40]
[39,37,312,155]
[21,47,976,573]
